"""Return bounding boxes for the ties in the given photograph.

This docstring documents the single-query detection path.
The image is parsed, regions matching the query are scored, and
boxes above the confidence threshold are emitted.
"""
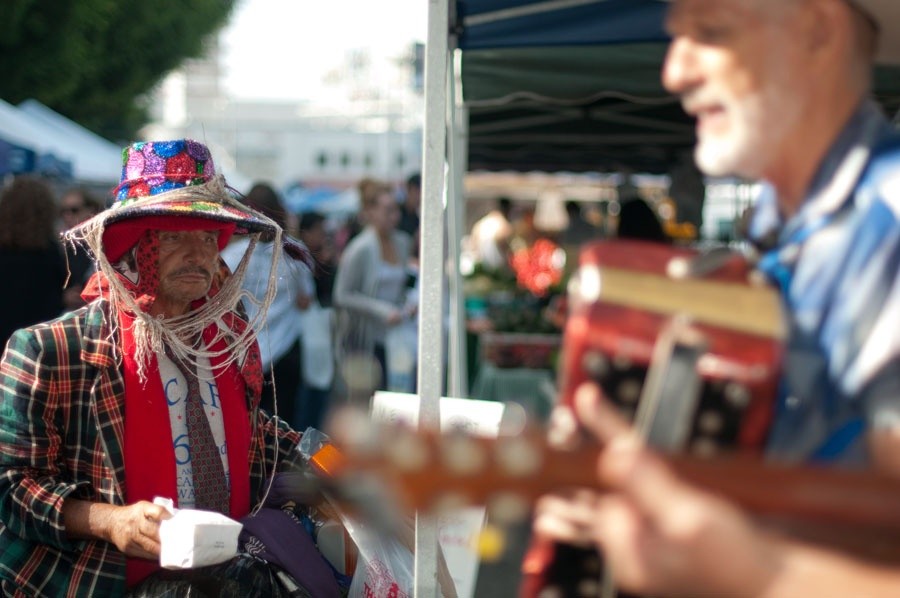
[158,323,232,517]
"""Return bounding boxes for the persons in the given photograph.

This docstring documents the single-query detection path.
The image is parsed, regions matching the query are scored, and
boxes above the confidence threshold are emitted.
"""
[0,181,765,434]
[0,140,342,598]
[574,0,900,598]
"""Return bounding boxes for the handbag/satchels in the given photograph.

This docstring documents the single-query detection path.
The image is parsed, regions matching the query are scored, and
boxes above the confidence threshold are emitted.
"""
[382,314,416,392]
[301,297,336,391]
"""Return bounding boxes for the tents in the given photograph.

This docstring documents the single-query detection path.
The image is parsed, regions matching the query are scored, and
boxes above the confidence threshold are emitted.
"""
[0,99,122,189]
[413,0,900,598]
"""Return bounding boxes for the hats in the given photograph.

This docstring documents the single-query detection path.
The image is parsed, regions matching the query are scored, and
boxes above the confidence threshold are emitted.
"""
[56,138,322,274]
